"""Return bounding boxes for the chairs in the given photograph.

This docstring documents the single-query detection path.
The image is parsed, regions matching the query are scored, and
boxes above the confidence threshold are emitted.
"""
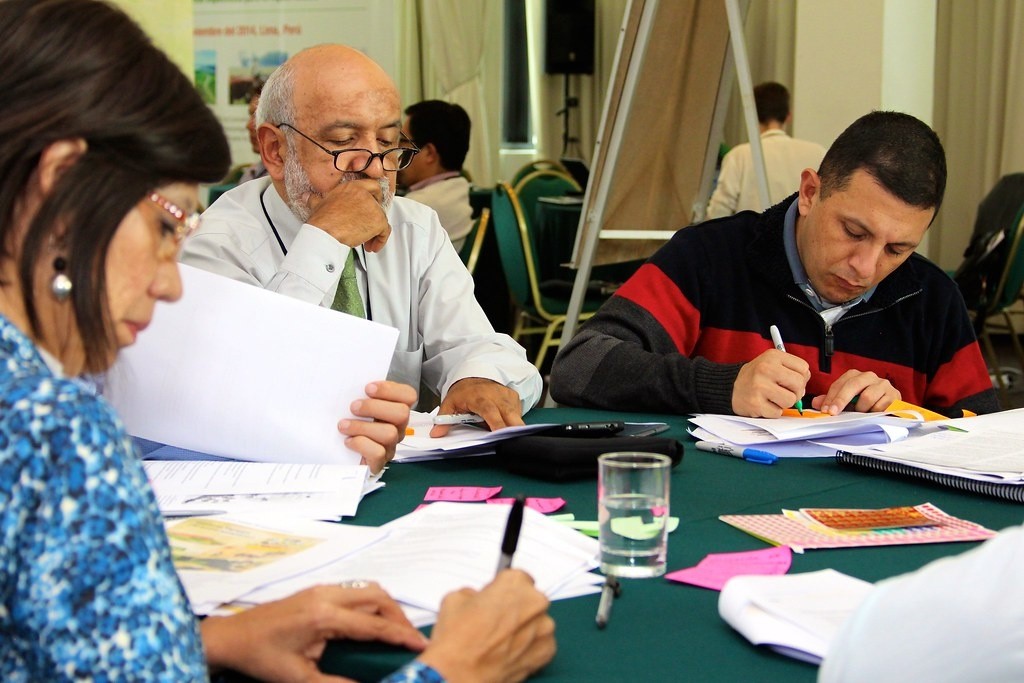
[942,203,1024,412]
[457,157,617,373]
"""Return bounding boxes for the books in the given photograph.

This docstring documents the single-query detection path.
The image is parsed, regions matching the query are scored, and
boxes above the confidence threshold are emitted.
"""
[834,408,1024,503]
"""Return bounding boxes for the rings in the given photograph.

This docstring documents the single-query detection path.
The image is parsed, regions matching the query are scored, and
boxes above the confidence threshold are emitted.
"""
[341,580,372,592]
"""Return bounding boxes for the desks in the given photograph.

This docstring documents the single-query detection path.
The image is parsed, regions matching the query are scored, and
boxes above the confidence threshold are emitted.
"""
[141,406,1024,683]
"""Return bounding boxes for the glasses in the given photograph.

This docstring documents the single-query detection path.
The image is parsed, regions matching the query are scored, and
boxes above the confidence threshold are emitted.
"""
[276,121,420,173]
[146,190,200,247]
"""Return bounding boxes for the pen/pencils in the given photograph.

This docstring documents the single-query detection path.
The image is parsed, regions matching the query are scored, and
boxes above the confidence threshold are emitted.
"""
[594,577,618,628]
[434,413,483,424]
[498,496,525,572]
[693,440,778,465]
[769,325,803,416]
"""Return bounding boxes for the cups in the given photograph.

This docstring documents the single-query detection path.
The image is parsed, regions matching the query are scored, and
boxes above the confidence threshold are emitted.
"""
[598,451,671,579]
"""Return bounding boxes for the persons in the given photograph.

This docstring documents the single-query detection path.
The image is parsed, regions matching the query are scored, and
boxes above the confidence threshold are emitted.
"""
[0,0,558,683]
[551,110,999,421]
[699,82,827,221]
[817,527,1024,683]
